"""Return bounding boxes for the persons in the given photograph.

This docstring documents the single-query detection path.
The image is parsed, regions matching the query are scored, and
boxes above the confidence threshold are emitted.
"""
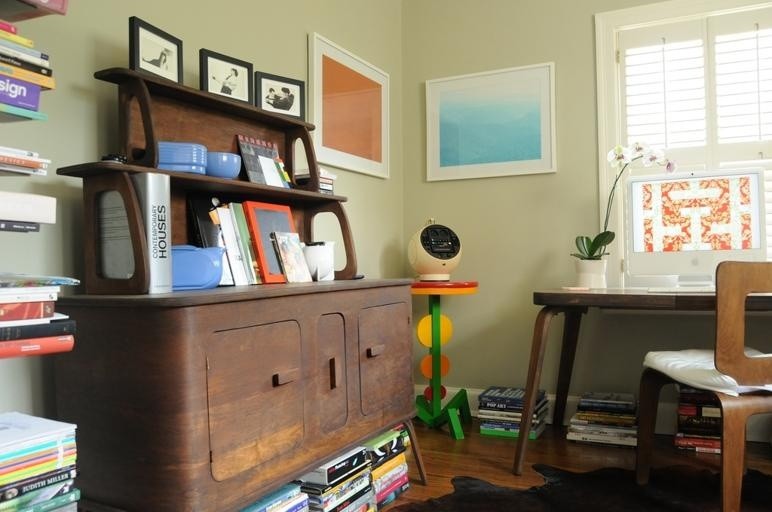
[143,47,173,70]
[265,88,280,100]
[268,87,290,110]
[212,68,238,95]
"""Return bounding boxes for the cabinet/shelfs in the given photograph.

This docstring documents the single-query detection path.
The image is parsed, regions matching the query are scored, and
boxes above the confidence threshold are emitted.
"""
[41,67,428,511]
[0,0,83,512]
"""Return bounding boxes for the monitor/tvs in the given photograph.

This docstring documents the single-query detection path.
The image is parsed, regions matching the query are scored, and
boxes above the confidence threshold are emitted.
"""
[625,167,766,285]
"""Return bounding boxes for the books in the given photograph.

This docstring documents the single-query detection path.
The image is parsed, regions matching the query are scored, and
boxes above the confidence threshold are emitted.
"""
[238,423,411,512]
[478,382,721,455]
[0,20,81,512]
[98,173,172,295]
[188,196,313,287]
[294,167,337,195]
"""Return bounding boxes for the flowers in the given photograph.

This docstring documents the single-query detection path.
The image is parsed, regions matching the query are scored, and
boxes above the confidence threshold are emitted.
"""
[568,140,676,261]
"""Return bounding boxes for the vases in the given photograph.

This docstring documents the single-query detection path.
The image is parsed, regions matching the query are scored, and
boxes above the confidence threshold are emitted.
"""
[575,260,607,292]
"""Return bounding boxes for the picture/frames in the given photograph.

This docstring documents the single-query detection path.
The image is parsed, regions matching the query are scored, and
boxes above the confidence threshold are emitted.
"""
[127,15,184,85]
[424,60,558,184]
[306,31,392,181]
[198,48,255,106]
[255,71,306,122]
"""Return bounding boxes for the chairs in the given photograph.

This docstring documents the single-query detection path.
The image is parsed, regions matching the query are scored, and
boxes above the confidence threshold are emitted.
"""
[635,259,772,511]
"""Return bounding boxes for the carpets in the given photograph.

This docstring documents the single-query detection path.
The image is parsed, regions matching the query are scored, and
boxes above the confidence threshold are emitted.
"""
[384,462,772,512]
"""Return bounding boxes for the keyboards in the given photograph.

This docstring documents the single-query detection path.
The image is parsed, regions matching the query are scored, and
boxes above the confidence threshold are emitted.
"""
[647,286,717,294]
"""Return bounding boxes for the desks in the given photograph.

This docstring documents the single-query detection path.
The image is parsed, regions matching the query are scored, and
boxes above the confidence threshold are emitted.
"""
[513,284,772,477]
[413,280,479,440]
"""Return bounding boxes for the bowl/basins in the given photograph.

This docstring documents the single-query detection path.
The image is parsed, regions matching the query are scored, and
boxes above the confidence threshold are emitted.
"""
[208,152,243,180]
